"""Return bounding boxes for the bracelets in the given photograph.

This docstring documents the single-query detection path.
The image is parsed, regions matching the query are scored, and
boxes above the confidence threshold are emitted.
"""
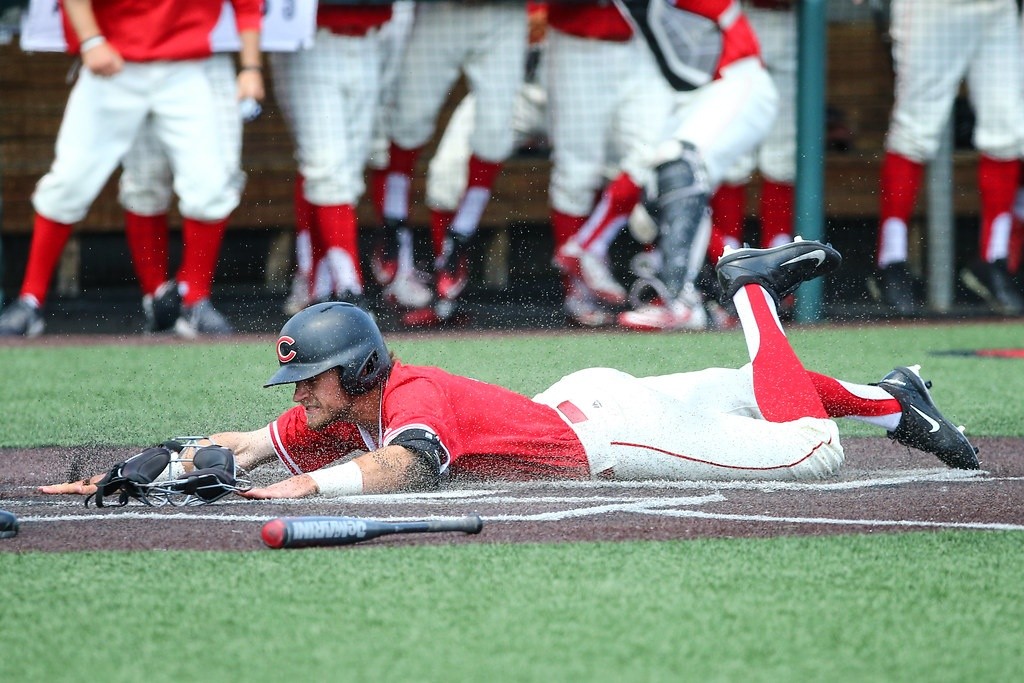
[241,65,264,72]
[80,34,106,53]
[305,460,364,495]
[143,452,186,480]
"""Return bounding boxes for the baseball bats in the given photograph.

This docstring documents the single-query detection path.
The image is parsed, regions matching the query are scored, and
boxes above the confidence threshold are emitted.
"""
[261,514,483,550]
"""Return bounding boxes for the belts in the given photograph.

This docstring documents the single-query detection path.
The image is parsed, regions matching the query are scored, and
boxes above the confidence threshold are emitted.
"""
[557,401,616,481]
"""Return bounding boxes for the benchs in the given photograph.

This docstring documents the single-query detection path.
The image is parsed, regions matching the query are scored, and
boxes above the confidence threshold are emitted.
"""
[0,0,1024,311]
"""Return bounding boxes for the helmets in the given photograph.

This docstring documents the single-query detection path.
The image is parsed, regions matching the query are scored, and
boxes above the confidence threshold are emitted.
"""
[263,302,392,395]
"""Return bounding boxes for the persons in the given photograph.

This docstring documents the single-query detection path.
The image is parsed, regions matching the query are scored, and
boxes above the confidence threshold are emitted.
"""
[34,238,980,498]
[879,0,1024,318]
[0,0,797,343]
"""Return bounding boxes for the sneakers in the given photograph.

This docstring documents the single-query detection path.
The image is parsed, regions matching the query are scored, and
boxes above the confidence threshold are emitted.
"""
[564,290,615,327]
[869,264,924,308]
[176,299,236,335]
[552,237,628,305]
[1,299,44,339]
[868,364,982,471]
[620,295,708,332]
[960,260,1023,314]
[715,236,842,307]
